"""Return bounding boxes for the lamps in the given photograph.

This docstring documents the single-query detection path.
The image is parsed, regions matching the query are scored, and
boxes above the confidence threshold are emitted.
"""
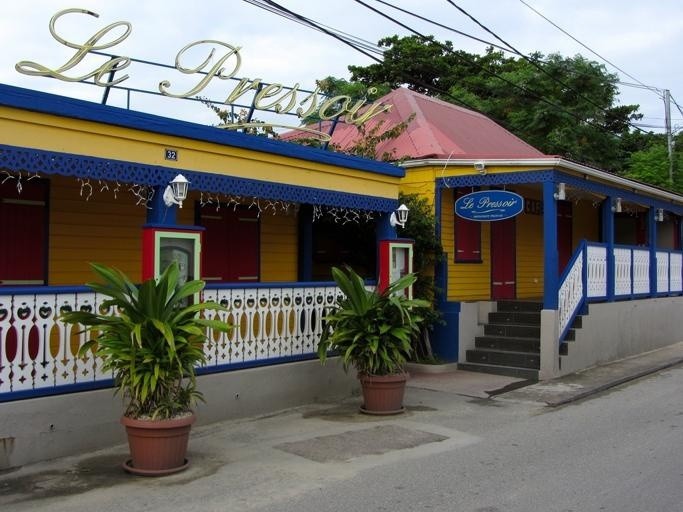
[611,197,622,212]
[163,173,192,208]
[654,208,664,222]
[554,183,566,200]
[389,203,410,228]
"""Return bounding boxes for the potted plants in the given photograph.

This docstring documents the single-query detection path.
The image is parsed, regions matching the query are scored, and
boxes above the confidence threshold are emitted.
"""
[53,258,242,477]
[316,265,433,415]
